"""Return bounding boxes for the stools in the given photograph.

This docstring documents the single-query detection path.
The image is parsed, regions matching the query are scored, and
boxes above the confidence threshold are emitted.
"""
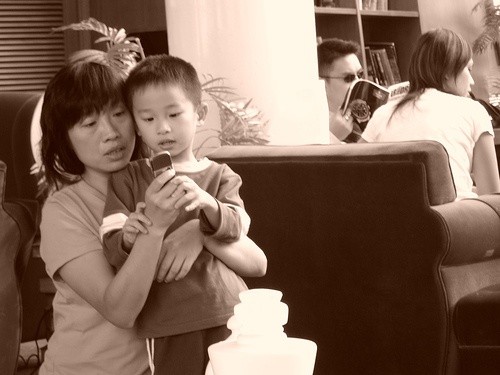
[452,284,500,375]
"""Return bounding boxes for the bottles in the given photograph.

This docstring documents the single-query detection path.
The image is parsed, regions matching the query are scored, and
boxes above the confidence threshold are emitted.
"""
[205,290,317,374]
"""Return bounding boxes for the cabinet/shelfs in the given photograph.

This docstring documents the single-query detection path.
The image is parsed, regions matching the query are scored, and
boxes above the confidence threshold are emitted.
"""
[314,0,423,89]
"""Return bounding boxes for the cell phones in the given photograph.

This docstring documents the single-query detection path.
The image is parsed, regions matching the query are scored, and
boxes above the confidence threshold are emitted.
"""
[150,151,175,186]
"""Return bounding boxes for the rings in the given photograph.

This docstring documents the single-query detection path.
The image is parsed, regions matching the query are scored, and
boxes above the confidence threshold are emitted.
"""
[343,116,350,121]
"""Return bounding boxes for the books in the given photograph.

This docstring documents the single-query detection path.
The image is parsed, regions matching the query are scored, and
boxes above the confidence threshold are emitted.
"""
[317,37,352,45]
[362,41,405,89]
[362,0,388,10]
[314,0,341,7]
[335,80,410,143]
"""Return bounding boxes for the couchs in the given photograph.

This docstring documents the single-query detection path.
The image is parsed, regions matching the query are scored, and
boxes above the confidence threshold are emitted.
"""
[204,140,500,375]
[0,90,56,375]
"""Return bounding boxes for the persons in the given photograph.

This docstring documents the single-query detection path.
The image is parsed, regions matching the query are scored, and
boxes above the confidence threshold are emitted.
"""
[317,37,365,143]
[98,53,254,375]
[37,61,267,375]
[357,27,500,199]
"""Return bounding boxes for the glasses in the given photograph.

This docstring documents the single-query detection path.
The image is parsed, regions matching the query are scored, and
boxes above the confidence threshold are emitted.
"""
[320,69,364,83]
